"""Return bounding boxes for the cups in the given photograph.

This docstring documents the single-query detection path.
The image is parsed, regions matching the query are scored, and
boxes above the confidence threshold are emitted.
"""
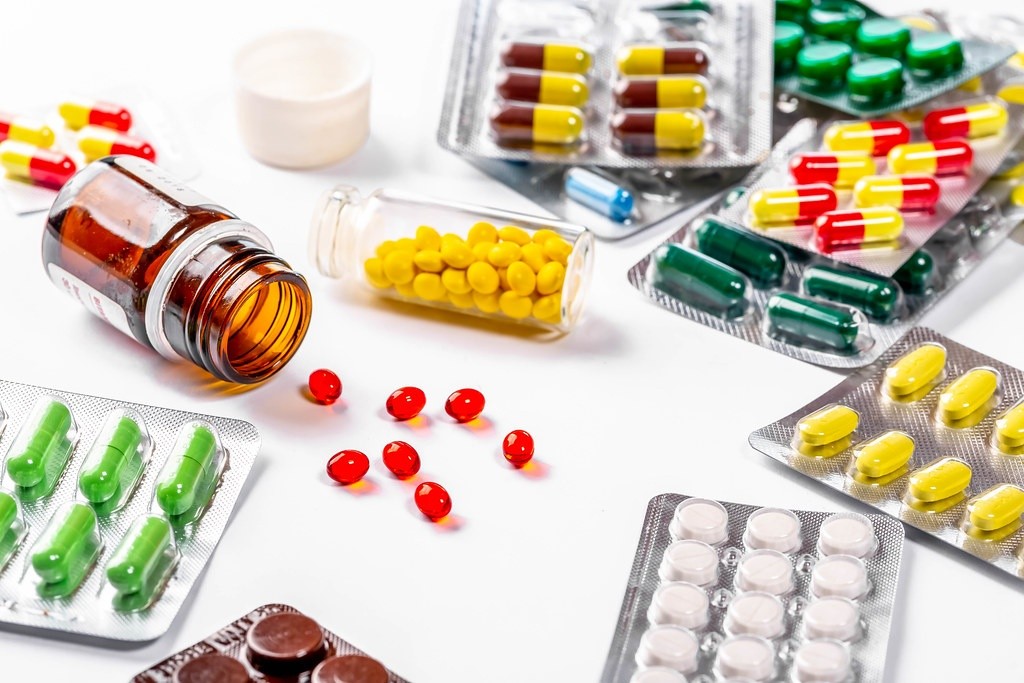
[227,25,375,168]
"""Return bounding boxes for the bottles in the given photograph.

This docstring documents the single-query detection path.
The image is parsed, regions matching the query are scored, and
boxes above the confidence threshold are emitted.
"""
[299,184,598,343]
[28,152,314,390]
